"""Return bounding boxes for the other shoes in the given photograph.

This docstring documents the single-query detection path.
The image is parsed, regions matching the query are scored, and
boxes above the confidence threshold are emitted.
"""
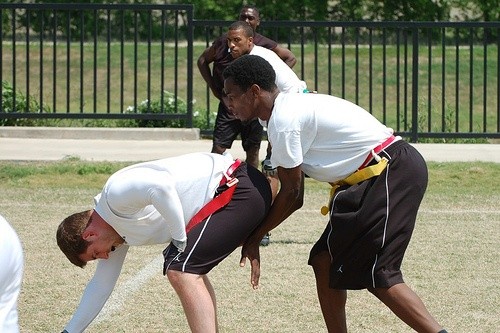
[260,232,270,246]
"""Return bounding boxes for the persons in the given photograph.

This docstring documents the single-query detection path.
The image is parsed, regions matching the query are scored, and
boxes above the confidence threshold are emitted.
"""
[56,151,272,333]
[222,54,447,333]
[197,7,297,170]
[228,21,309,246]
[0,214,25,333]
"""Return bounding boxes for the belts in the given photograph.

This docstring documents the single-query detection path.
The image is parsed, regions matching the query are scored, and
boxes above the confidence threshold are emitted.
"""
[219,158,241,187]
[355,134,396,173]
